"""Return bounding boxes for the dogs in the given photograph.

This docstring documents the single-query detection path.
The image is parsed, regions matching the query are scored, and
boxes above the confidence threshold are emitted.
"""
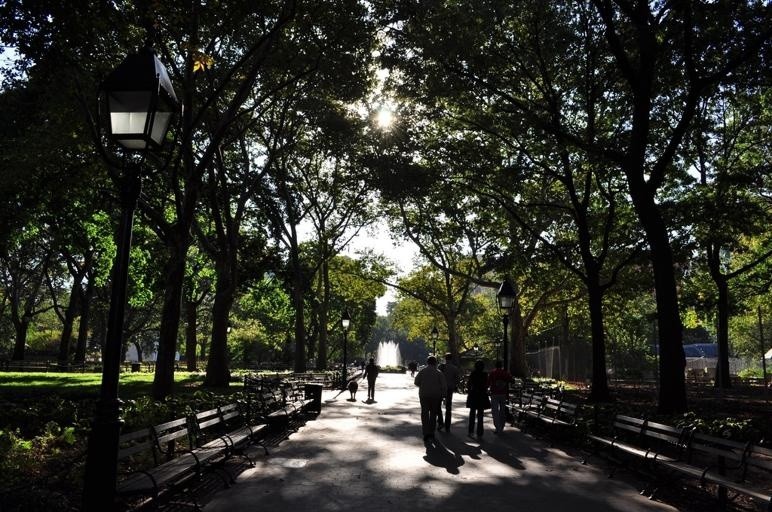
[348,381,359,401]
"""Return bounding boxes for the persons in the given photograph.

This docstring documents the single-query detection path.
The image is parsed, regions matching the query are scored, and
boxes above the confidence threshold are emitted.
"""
[363,358,378,400]
[489,361,515,434]
[466,361,491,437]
[416,357,448,441]
[436,352,457,432]
[410,359,416,377]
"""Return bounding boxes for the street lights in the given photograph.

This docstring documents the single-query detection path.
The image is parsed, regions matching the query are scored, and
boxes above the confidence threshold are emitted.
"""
[341,307,351,390]
[473,343,479,361]
[431,326,439,356]
[495,280,516,371]
[78,45,181,512]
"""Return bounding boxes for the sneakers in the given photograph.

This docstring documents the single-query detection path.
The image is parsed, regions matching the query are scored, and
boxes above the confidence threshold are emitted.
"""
[437,423,451,434]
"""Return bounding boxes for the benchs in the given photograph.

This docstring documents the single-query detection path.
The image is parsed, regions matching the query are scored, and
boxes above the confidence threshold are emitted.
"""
[586,405,772,512]
[116,403,270,511]
[507,390,579,439]
[260,388,315,438]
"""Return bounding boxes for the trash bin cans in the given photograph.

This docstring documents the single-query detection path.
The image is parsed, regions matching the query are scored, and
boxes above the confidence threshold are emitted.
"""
[131,363,141,372]
[304,383,323,413]
